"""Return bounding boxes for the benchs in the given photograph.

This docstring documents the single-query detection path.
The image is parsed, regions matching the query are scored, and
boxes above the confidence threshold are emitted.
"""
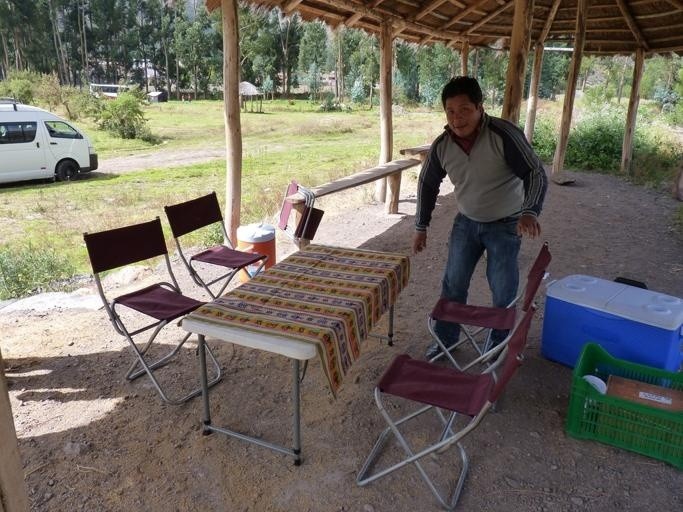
[285,139,431,245]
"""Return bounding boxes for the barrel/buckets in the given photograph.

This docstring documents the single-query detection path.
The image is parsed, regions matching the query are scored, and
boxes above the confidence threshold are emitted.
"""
[237,221,275,282]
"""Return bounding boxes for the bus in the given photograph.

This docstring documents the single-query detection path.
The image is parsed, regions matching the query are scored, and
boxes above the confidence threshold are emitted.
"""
[90,84,130,99]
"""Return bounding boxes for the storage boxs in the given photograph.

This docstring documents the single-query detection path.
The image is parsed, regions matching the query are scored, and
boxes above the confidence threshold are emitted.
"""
[538,274,682,371]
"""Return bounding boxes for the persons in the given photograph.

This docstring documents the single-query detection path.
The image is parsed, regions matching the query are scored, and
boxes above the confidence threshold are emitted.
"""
[411,77,547,364]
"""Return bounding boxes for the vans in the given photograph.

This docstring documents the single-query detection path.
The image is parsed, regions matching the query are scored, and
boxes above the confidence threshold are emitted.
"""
[0,97,98,185]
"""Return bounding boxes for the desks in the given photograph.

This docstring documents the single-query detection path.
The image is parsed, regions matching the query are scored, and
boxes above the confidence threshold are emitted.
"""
[176,244,409,466]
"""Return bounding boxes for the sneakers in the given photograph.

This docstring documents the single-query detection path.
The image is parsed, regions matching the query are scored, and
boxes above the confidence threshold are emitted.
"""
[425,343,456,362]
[486,340,505,363]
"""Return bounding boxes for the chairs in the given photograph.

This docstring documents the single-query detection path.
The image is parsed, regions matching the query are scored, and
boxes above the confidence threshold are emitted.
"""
[164,191,268,300]
[358,298,539,511]
[82,215,225,407]
[425,238,552,373]
